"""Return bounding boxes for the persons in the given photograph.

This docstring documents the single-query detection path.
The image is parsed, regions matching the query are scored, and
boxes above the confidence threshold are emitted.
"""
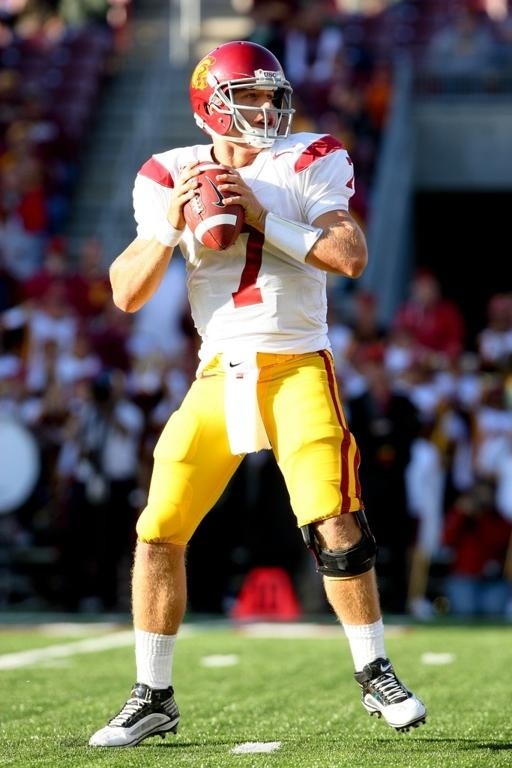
[87,40,429,749]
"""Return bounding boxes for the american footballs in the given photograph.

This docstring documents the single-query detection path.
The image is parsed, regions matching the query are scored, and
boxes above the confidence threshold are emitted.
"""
[181,161,244,252]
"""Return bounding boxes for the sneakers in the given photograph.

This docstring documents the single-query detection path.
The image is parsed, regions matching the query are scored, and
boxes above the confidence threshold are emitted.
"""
[355,658,426,732]
[89,683,180,746]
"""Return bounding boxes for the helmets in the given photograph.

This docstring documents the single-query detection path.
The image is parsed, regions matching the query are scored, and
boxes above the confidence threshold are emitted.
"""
[190,41,294,141]
